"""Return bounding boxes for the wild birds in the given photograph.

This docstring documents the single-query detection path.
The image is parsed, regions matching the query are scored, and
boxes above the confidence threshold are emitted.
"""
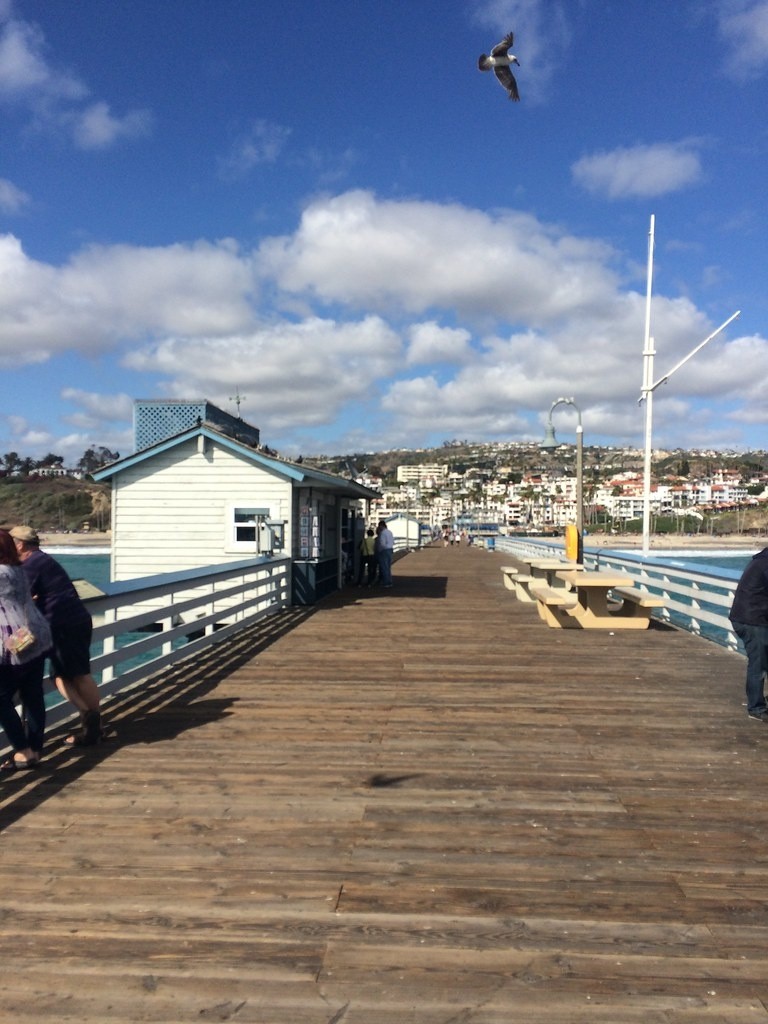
[477,31,522,104]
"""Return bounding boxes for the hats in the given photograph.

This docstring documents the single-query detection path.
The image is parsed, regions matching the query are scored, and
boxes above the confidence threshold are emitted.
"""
[9,525,37,541]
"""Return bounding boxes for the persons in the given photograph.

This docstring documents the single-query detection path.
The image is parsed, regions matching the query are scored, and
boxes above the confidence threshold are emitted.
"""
[360,530,377,582]
[0,529,54,768]
[727,544,768,722]
[444,532,448,547]
[374,520,393,588]
[9,525,107,744]
[455,534,460,544]
[450,533,455,545]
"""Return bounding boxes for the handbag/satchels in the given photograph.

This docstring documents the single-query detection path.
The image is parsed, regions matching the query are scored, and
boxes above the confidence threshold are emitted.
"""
[4,625,35,656]
[367,559,377,568]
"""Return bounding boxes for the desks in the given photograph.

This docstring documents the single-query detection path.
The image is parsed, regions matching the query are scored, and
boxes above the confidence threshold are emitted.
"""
[524,558,560,579]
[529,562,584,592]
[555,570,634,629]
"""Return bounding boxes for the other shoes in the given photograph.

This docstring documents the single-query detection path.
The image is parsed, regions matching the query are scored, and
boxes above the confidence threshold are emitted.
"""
[749,709,768,722]
[63,712,108,745]
[384,583,392,588]
[2,753,36,768]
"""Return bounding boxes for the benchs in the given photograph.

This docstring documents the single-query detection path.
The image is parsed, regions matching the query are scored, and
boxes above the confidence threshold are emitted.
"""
[510,573,538,602]
[613,586,666,629]
[500,566,518,590]
[531,587,573,628]
